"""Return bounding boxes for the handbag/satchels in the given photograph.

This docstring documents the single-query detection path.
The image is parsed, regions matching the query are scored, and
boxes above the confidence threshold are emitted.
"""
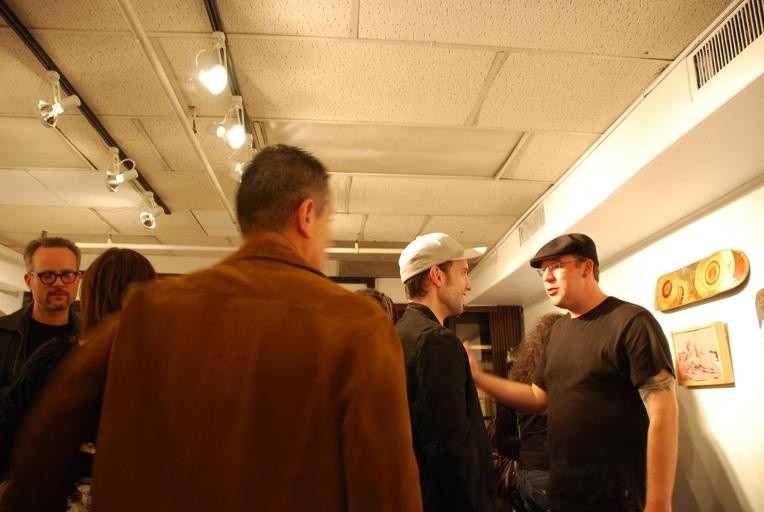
[487,418,520,499]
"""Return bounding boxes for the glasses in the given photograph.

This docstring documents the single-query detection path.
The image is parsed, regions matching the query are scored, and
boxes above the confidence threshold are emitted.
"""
[30,270,78,284]
[536,260,578,276]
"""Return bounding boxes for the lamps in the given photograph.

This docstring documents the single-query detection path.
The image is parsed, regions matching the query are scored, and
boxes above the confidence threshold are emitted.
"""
[194,1,270,183]
[0,1,173,230]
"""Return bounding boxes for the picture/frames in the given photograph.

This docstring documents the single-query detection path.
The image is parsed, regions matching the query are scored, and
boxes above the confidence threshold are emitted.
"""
[670,321,734,387]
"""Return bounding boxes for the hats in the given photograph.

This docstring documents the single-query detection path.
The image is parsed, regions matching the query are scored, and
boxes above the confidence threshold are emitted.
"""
[530,233,599,268]
[398,233,488,283]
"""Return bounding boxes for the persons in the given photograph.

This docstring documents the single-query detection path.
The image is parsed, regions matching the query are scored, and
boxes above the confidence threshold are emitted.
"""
[456,234,679,511]
[1,235,161,511]
[350,232,493,512]
[0,145,425,512]
